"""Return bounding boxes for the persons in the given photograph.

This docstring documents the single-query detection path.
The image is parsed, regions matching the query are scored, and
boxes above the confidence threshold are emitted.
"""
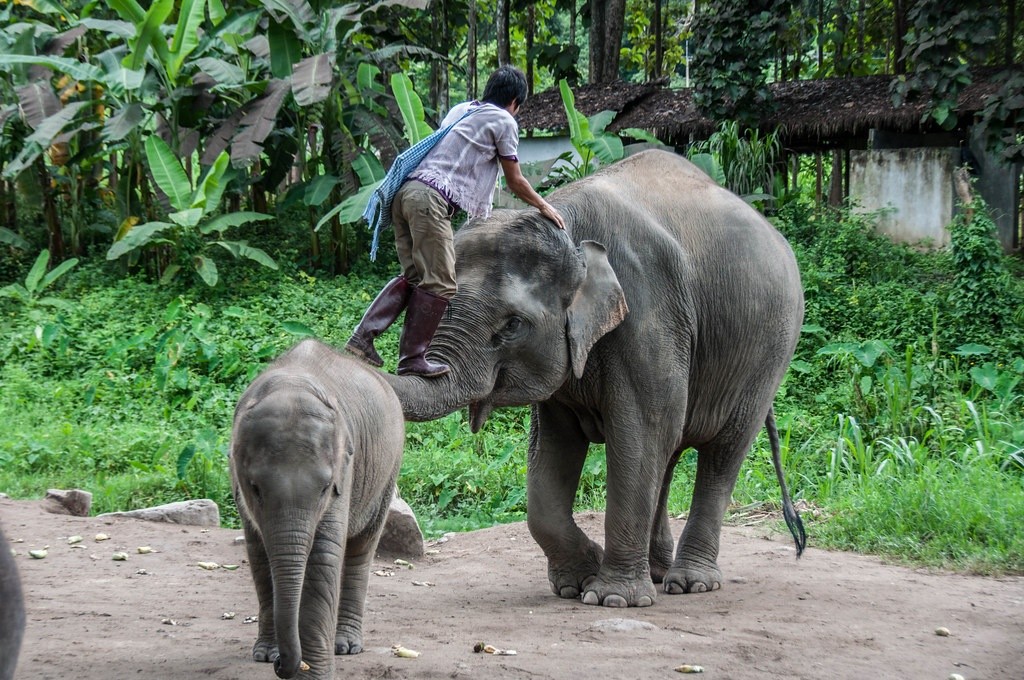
[344,66,566,377]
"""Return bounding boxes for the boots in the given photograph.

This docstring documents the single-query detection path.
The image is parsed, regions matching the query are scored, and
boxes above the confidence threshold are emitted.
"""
[396,287,451,378]
[344,273,416,368]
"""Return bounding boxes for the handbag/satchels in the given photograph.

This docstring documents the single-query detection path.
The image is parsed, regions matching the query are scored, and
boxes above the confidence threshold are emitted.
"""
[363,105,480,263]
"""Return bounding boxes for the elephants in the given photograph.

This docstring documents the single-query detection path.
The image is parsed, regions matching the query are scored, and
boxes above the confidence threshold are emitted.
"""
[371,148,809,608]
[229,339,406,679]
